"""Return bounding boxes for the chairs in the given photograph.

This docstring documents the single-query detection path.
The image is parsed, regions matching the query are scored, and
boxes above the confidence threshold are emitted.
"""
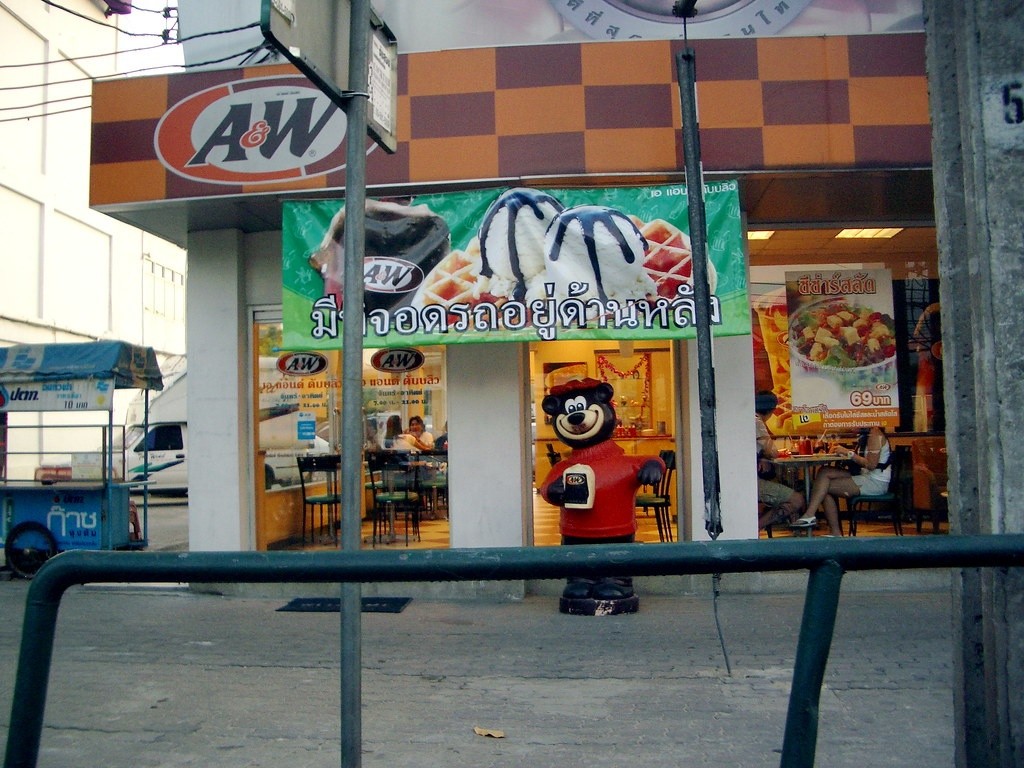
[847,445,907,534]
[297,448,449,549]
[634,449,676,542]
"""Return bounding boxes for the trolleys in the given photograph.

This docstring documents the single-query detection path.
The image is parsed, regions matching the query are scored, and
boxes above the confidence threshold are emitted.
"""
[0,341,164,581]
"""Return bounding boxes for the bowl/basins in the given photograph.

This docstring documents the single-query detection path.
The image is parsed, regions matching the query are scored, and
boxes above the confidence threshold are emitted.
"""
[788,294,897,392]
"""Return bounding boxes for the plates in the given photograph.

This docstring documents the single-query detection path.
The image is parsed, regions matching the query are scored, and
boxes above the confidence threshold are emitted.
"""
[790,455,812,458]
[813,454,836,456]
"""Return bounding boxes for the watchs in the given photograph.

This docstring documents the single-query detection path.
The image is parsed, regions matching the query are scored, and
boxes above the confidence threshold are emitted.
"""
[848,451,854,457]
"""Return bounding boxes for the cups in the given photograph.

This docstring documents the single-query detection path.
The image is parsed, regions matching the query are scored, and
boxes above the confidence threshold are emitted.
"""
[657,420,665,436]
[777,449,786,457]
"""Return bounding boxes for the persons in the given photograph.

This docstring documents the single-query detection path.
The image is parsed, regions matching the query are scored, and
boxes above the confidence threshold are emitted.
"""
[406,416,435,450]
[791,426,891,538]
[380,415,430,492]
[755,390,804,531]
[436,421,448,449]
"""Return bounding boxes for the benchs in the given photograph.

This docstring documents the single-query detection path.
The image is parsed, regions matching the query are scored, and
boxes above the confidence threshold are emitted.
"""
[912,437,948,534]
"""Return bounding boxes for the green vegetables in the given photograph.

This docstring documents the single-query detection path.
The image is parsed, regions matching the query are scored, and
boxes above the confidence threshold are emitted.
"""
[793,303,899,368]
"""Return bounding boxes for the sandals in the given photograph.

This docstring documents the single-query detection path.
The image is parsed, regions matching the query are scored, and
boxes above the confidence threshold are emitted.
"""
[790,516,817,527]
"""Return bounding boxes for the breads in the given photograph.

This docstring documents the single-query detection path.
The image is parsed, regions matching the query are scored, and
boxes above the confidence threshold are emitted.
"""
[545,364,587,387]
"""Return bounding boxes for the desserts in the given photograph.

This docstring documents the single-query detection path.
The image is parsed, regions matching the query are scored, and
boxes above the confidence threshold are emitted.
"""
[412,187,717,328]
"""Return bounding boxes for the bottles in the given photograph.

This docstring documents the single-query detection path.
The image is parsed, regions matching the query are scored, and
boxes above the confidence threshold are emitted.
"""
[636,418,641,437]
[799,435,811,455]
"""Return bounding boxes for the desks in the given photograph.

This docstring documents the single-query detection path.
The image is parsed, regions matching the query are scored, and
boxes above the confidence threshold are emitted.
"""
[339,460,427,536]
[761,456,852,536]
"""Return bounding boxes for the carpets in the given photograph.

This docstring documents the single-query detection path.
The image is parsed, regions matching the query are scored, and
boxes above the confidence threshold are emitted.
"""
[275,597,416,613]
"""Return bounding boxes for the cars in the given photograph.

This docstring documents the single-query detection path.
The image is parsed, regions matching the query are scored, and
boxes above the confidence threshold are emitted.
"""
[102,422,187,494]
